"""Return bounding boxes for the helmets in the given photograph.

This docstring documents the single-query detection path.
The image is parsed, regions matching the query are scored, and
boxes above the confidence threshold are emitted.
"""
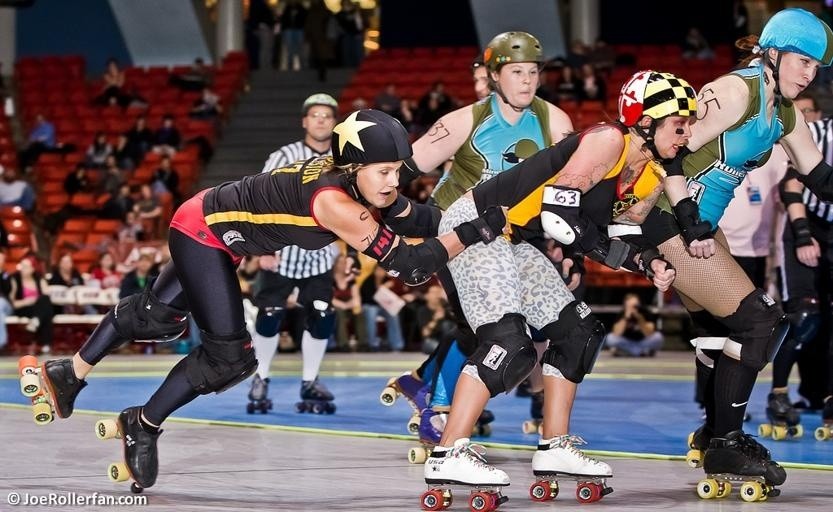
[331,108,412,166]
[758,8,833,68]
[617,69,698,126]
[483,31,545,72]
[302,93,338,117]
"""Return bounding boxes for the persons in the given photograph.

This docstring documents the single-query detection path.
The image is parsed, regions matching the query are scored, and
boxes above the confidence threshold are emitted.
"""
[0,30,833,357]
[644,8,832,488]
[742,355,833,424]
[393,358,614,483]
[246,0,378,32]
[242,94,348,415]
[15,109,513,493]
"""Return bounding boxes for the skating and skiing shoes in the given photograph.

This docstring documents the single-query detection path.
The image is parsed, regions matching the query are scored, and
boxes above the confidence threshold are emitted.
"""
[379,369,498,464]
[18,355,88,425]
[531,436,614,503]
[522,388,546,434]
[686,421,786,502]
[247,374,272,415]
[420,439,509,512]
[94,406,163,493]
[759,394,832,439]
[294,375,336,414]
